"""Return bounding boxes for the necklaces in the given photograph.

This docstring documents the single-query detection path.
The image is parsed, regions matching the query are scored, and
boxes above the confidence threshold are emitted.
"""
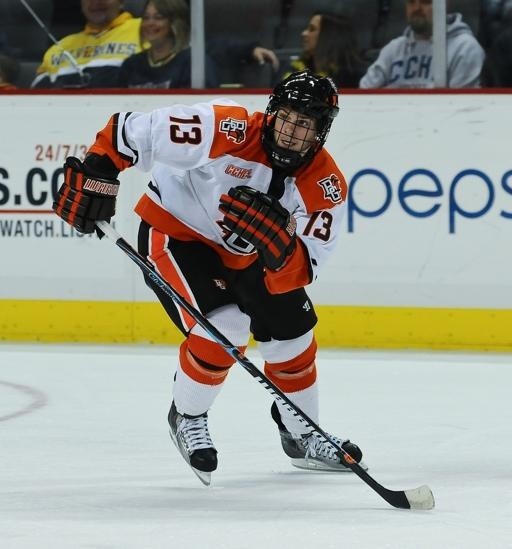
[146,46,178,67]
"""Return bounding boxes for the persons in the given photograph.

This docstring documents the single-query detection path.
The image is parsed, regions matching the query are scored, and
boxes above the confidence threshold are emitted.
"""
[286,11,369,89]
[51,72,370,486]
[359,0,485,89]
[109,2,224,89]
[28,1,281,88]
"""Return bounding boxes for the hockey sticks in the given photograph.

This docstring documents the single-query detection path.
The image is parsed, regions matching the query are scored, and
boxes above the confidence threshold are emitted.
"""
[93,218,436,509]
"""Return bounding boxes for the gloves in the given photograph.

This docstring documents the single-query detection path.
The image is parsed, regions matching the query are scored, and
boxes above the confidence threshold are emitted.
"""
[215,185,298,272]
[52,156,121,242]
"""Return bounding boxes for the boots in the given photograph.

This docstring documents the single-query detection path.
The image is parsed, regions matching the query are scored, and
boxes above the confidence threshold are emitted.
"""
[166,399,219,472]
[271,401,363,468]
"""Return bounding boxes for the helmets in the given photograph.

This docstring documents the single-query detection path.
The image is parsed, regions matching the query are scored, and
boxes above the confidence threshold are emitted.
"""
[262,71,340,170]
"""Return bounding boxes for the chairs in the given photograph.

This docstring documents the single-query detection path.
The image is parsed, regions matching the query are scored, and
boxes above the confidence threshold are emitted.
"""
[0,0,511,97]
